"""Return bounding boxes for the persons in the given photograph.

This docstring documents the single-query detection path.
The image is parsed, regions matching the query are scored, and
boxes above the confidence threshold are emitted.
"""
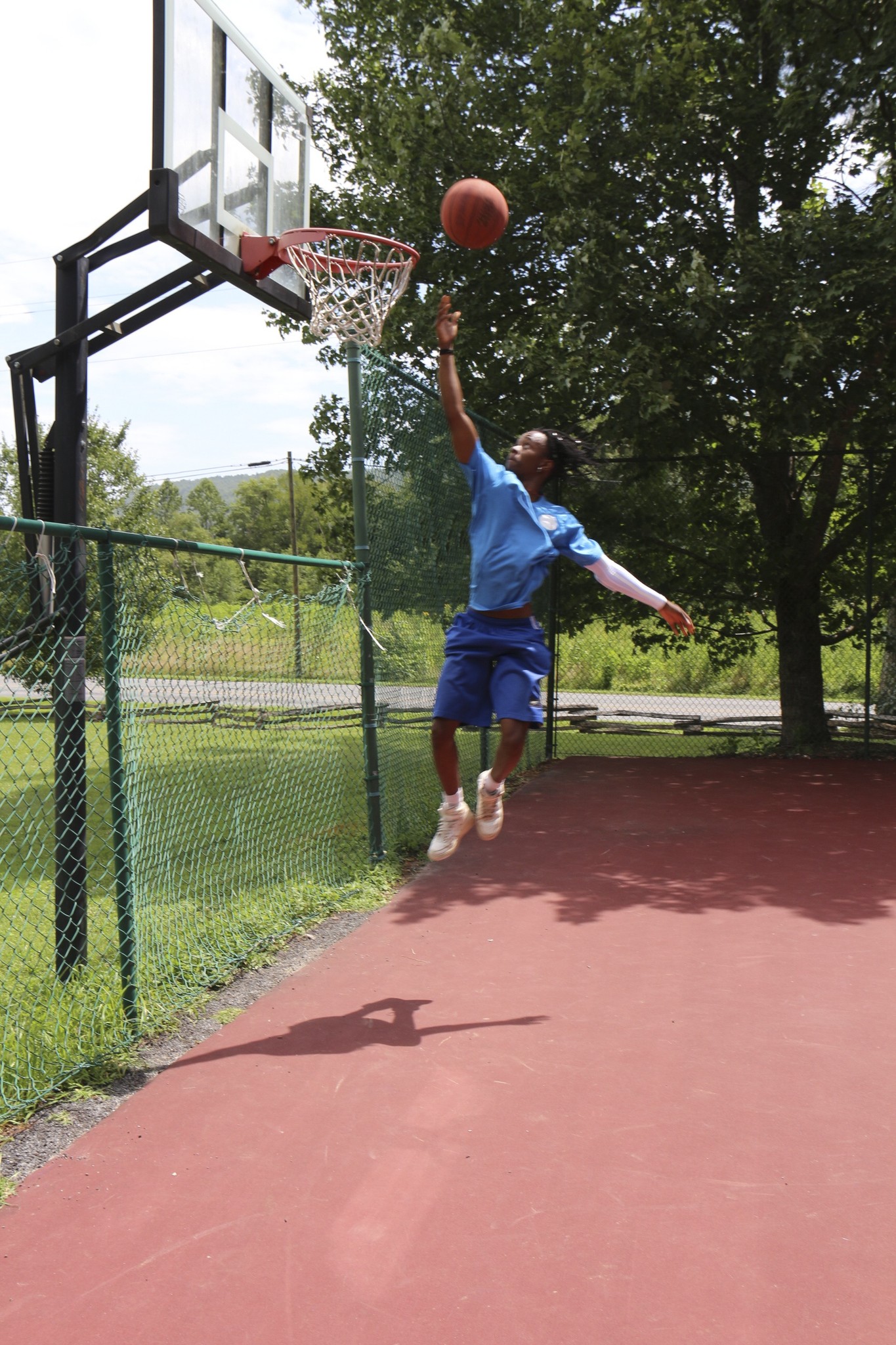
[425,293,695,863]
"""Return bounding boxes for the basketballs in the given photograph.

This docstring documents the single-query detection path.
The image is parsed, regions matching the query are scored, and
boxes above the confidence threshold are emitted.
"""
[441,178,509,250]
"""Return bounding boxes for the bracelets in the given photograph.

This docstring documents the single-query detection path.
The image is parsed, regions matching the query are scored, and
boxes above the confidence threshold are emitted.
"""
[439,348,456,354]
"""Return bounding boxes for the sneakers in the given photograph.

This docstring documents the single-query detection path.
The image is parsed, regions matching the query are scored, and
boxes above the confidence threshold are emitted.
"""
[475,770,505,842]
[427,802,474,861]
